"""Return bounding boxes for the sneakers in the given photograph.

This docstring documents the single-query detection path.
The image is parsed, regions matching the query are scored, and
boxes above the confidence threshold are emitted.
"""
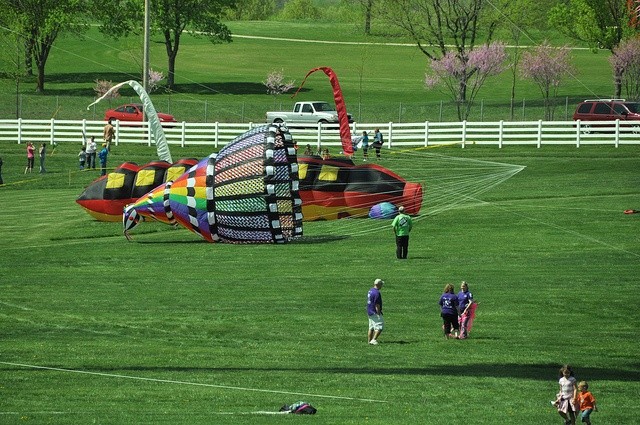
[369,337,379,345]
[564,420,572,425]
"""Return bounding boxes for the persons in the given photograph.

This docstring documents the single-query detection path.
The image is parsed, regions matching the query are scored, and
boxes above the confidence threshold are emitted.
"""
[314,146,323,157]
[572,381,599,425]
[98,143,110,177]
[293,140,298,154]
[304,142,313,155]
[372,126,383,160]
[453,282,476,337]
[23,140,35,174]
[102,119,116,152]
[392,205,413,258]
[320,147,330,159]
[37,142,47,174]
[550,362,580,425]
[439,283,462,339]
[366,278,386,344]
[77,145,87,170]
[85,135,97,169]
[360,130,371,162]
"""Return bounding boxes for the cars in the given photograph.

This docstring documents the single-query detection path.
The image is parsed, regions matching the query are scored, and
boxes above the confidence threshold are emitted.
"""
[104,103,175,127]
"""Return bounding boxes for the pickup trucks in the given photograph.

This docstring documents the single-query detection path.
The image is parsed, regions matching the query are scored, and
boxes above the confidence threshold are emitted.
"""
[266,100,355,130]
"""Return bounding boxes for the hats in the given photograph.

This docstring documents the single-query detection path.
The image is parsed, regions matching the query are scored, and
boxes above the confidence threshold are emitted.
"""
[374,279,385,285]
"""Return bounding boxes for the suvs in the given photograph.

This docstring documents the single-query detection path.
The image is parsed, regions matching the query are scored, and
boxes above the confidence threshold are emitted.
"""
[573,98,640,134]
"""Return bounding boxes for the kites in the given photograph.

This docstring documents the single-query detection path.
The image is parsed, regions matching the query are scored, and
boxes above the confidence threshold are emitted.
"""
[121,120,306,249]
[442,301,483,336]
[73,66,425,227]
